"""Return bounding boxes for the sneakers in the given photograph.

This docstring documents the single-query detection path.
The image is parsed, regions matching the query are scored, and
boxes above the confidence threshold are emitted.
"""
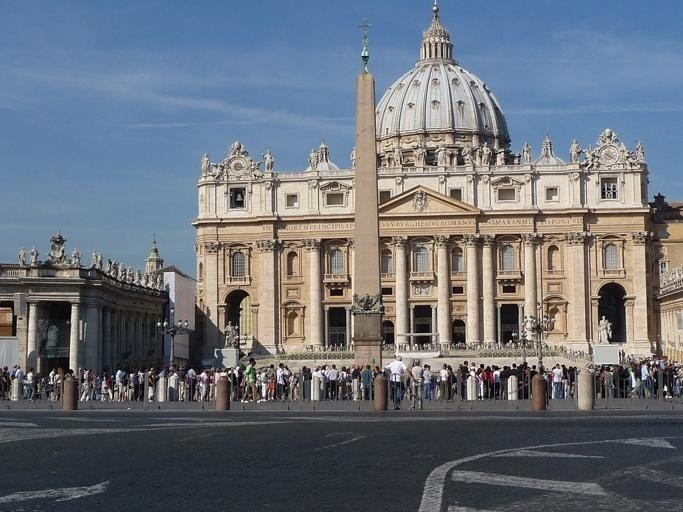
[240,397,275,406]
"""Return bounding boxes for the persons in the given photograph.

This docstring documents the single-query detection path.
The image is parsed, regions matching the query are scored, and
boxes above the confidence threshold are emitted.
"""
[586,356,683,398]
[214,161,228,180]
[597,314,609,343]
[241,357,312,403]
[623,150,634,171]
[313,364,383,401]
[479,142,492,165]
[434,141,446,166]
[223,320,234,347]
[0,364,74,401]
[659,265,683,290]
[80,364,241,402]
[522,142,531,163]
[580,148,591,171]
[262,149,273,172]
[637,143,644,162]
[392,145,403,166]
[250,161,262,179]
[570,140,581,162]
[199,153,210,176]
[350,146,356,168]
[415,141,427,166]
[359,293,372,310]
[308,148,318,170]
[456,361,577,400]
[460,143,472,164]
[385,357,456,408]
[18,243,163,290]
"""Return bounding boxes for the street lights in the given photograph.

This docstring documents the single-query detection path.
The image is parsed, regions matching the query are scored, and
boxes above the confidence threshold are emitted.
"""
[156,307,189,376]
[528,304,555,369]
[507,322,531,362]
